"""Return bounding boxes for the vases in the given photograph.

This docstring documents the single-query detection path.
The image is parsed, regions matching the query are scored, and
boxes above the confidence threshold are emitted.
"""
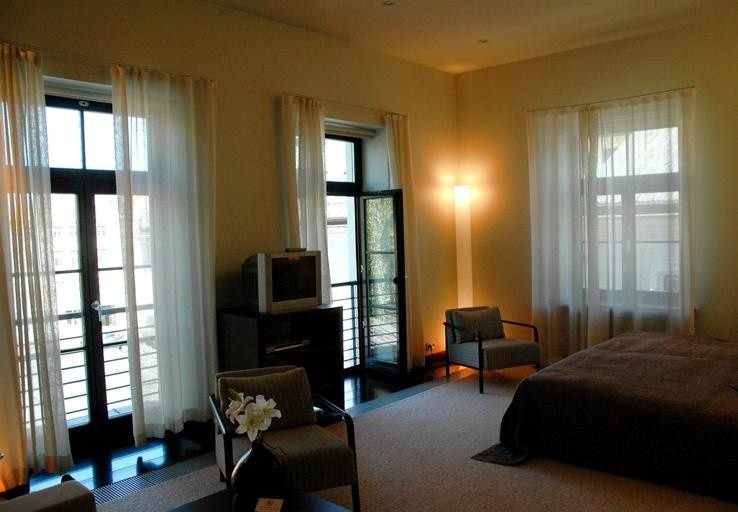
[232,438,275,485]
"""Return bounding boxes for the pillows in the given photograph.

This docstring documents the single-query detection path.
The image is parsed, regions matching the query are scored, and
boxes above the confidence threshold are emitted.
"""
[452,307,505,344]
[215,367,318,427]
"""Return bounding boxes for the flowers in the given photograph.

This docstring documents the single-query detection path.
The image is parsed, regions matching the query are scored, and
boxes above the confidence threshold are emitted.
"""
[225,394,284,490]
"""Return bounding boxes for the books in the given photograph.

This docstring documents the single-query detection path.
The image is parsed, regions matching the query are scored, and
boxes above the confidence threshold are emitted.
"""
[255,497,283,512]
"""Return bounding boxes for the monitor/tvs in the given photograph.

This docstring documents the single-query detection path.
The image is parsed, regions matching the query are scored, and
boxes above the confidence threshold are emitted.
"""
[241,250,322,315]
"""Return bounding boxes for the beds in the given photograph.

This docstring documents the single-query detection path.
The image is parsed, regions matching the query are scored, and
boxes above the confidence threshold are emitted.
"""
[471,330,738,475]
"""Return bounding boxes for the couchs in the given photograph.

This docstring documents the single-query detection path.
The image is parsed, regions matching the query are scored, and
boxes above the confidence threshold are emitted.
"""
[204,365,362,512]
[444,305,540,393]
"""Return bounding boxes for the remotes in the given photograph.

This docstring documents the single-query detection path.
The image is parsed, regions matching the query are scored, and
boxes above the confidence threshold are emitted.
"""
[285,248,306,253]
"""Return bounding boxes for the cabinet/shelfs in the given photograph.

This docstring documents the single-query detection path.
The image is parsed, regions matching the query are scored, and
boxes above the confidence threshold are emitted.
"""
[219,307,343,418]
